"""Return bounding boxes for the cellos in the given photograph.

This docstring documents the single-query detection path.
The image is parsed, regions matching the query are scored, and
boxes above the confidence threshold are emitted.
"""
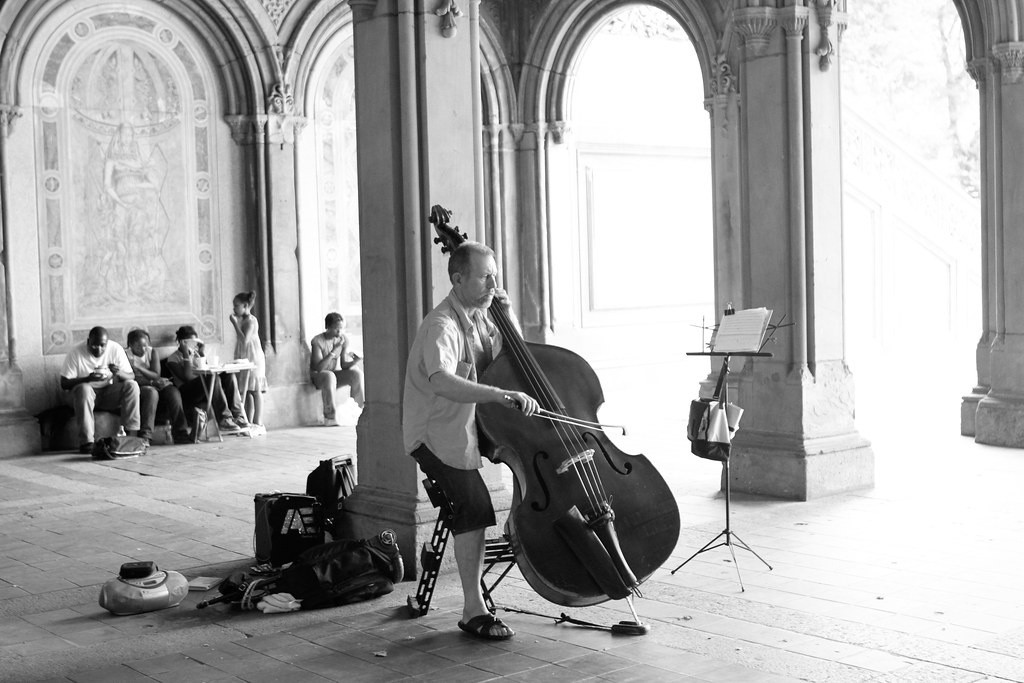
[428,203,681,624]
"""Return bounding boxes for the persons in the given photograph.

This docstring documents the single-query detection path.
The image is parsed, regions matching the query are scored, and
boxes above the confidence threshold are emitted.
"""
[60,326,141,453]
[229,290,269,439]
[310,312,364,427]
[123,329,193,445]
[402,242,541,641]
[166,325,253,431]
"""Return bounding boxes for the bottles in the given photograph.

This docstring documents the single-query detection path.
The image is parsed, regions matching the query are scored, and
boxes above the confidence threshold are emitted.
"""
[117,426,126,437]
[165,421,172,444]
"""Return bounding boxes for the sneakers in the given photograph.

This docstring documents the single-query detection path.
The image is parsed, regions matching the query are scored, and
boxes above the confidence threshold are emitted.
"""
[245,424,266,435]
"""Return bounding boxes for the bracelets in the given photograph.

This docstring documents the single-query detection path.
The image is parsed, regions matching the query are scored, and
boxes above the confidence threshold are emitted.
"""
[329,352,335,356]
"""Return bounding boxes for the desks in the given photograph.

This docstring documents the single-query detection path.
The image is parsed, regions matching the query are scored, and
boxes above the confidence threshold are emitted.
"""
[193,364,257,442]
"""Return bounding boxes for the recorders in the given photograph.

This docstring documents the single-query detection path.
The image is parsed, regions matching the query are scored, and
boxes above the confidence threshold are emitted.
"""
[99,571,189,616]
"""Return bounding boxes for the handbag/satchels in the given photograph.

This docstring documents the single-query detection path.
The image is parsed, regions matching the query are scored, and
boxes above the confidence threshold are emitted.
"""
[687,361,743,461]
[94,435,147,459]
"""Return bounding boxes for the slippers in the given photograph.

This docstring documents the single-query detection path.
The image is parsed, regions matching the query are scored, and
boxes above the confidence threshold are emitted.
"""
[458,613,512,639]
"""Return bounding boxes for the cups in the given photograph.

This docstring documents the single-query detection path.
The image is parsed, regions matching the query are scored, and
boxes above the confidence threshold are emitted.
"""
[208,357,219,367]
[95,368,109,378]
[197,357,206,367]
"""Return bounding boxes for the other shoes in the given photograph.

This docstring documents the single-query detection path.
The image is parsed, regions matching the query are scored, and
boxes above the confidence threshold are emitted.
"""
[174,433,193,442]
[324,418,339,426]
[80,444,94,454]
[143,437,151,447]
[220,419,241,430]
[235,418,249,427]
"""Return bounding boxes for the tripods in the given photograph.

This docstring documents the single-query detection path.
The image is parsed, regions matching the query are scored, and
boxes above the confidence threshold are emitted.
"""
[671,313,797,592]
